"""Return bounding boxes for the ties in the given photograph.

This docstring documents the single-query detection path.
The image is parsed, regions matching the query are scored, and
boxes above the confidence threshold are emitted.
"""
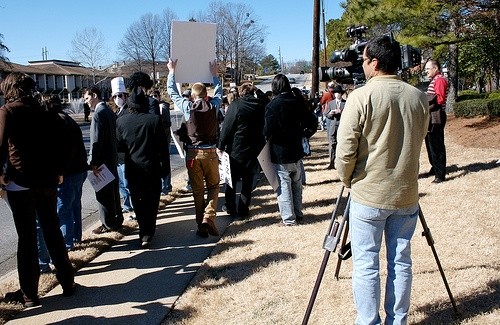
[336,100,340,109]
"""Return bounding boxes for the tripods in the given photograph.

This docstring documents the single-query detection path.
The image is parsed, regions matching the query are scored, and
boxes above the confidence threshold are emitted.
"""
[302,181,462,325]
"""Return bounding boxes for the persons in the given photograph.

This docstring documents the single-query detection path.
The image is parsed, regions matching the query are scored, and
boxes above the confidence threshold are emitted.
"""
[167,58,222,238]
[220,84,346,211]
[265,74,318,224]
[422,60,449,182]
[84,77,172,246]
[0,72,88,307]
[218,83,269,219]
[334,35,431,325]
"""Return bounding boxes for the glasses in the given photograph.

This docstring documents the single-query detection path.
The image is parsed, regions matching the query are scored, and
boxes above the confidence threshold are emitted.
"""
[113,94,123,98]
[182,94,190,98]
[149,95,159,98]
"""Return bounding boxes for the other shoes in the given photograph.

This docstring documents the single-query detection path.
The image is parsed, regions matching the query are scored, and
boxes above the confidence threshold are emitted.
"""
[139,237,153,246]
[122,208,133,213]
[202,218,220,236]
[160,188,172,196]
[92,223,111,234]
[431,177,446,183]
[280,217,304,226]
[40,266,52,273]
[197,229,209,238]
[424,169,435,176]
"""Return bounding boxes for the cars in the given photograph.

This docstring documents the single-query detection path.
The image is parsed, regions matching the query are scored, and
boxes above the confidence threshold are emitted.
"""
[300,70,304,75]
[288,77,296,84]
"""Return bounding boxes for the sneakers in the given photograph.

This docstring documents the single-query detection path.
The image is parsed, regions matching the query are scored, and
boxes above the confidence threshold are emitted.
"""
[4,288,40,307]
[62,282,89,296]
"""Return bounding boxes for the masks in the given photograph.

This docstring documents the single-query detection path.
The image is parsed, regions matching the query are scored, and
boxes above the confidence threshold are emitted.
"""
[114,96,124,107]
[184,98,190,101]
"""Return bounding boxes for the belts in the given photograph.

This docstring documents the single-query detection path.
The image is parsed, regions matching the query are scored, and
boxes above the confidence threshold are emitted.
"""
[187,148,216,153]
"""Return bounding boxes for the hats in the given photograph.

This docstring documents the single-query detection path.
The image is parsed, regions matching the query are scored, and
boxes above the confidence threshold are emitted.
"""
[127,88,150,109]
[331,85,346,96]
[182,90,191,96]
[327,82,336,87]
[110,76,127,97]
